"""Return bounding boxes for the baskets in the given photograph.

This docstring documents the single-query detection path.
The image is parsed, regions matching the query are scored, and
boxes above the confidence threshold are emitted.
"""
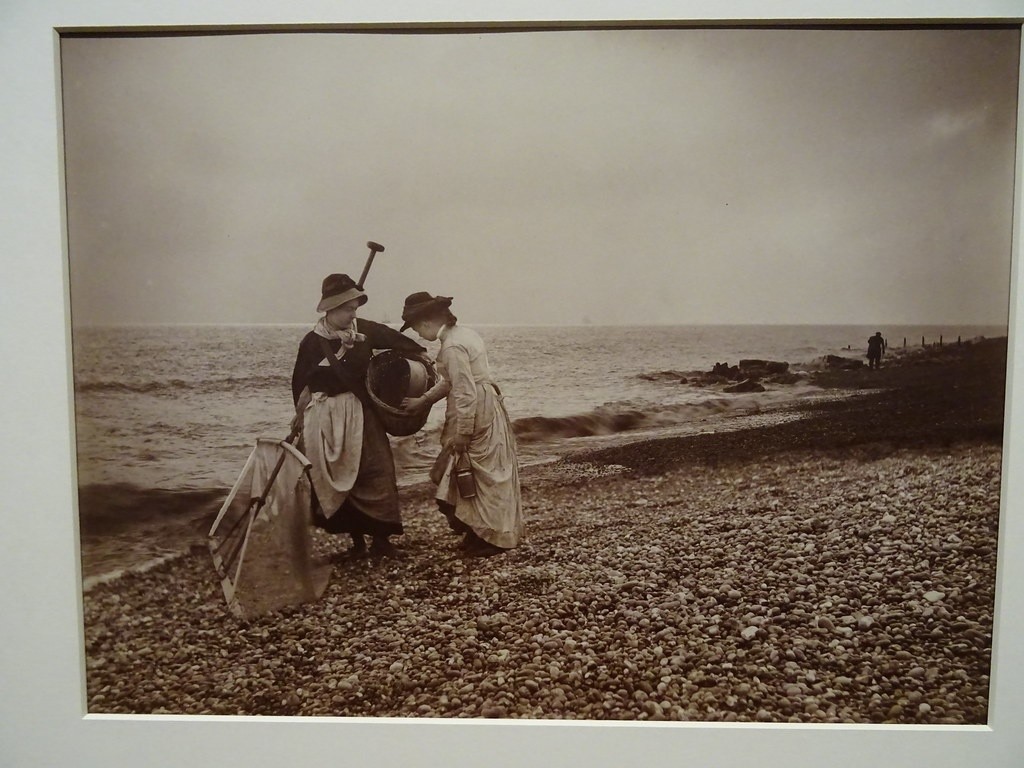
[365,348,441,437]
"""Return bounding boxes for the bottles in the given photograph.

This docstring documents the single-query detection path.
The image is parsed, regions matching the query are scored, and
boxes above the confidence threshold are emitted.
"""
[457,468,476,499]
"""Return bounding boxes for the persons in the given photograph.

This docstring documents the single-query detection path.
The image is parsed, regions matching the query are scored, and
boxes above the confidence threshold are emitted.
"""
[866,332,885,370]
[292,273,524,555]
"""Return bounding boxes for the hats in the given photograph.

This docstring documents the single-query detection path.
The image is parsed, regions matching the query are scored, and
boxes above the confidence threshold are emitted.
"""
[317,273,368,312]
[399,291,454,332]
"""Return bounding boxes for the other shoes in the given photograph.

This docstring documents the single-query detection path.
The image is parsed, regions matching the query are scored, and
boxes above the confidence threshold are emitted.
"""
[350,536,368,561]
[371,539,410,558]
[463,542,487,554]
[464,548,502,558]
